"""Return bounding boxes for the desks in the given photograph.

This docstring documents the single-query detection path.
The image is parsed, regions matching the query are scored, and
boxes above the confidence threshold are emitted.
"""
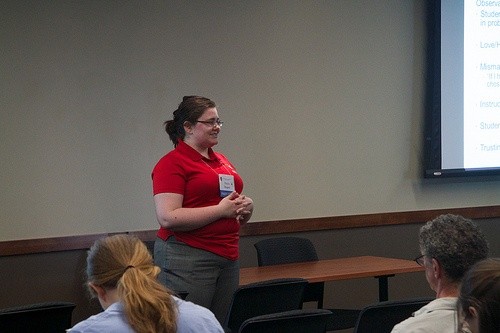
[240,256,424,333]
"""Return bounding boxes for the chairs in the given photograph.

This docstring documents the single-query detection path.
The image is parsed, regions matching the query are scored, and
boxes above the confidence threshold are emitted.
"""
[0,302,77,333]
[228,236,435,333]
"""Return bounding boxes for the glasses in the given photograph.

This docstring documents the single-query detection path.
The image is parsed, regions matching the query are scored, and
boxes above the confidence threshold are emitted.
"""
[415,254,424,268]
[191,118,224,127]
[456,315,472,333]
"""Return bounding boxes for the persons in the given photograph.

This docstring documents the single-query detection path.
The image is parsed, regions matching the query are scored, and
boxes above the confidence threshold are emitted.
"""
[391,213,488,333]
[66,234,225,333]
[454,258,500,333]
[152,96,254,333]
